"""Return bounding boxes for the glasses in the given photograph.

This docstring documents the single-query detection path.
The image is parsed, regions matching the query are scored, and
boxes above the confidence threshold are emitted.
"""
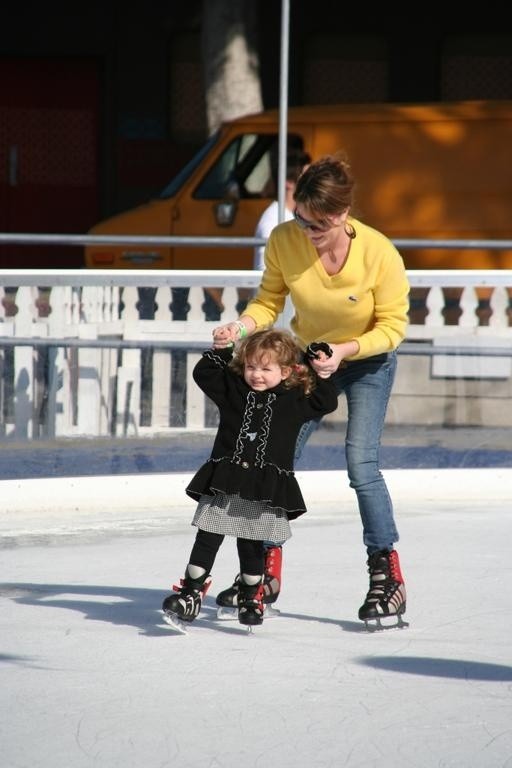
[292,207,336,232]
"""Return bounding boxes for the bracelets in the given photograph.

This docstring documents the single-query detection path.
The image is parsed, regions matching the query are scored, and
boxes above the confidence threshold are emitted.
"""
[234,319,249,339]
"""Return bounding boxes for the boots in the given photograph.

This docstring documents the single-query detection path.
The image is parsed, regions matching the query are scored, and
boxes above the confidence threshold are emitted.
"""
[234,572,265,626]
[359,549,406,617]
[161,563,213,623]
[216,545,281,606]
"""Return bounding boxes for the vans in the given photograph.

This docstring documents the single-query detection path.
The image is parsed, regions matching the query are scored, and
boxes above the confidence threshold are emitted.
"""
[82,100,510,328]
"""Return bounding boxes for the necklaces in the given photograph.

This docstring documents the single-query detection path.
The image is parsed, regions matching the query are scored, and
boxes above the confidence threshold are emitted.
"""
[319,232,341,266]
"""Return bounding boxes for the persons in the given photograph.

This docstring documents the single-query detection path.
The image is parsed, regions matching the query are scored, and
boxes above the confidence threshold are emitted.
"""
[208,152,412,621]
[248,149,316,337]
[157,325,339,631]
